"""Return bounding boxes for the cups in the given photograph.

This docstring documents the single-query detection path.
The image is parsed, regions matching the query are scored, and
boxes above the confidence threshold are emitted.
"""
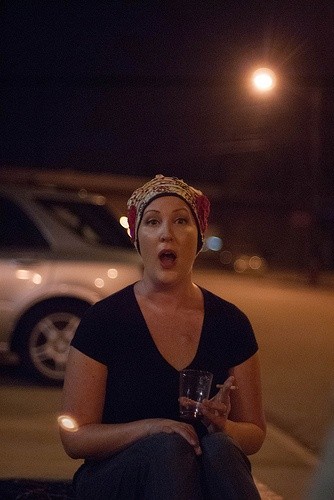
[179,368,212,421]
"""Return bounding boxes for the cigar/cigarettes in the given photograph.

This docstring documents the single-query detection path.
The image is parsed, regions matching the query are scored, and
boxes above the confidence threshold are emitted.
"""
[217,385,239,391]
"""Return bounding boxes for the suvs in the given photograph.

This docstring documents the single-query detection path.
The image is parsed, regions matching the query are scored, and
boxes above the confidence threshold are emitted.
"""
[0,189,143,386]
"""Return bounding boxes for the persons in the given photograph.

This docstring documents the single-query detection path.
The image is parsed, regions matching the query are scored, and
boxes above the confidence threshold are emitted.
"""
[59,173,268,500]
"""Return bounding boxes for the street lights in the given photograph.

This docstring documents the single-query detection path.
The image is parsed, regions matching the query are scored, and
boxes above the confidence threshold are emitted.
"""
[256,67,319,285]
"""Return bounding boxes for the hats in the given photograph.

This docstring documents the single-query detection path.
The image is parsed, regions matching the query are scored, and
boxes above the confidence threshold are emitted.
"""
[125,176,210,257]
